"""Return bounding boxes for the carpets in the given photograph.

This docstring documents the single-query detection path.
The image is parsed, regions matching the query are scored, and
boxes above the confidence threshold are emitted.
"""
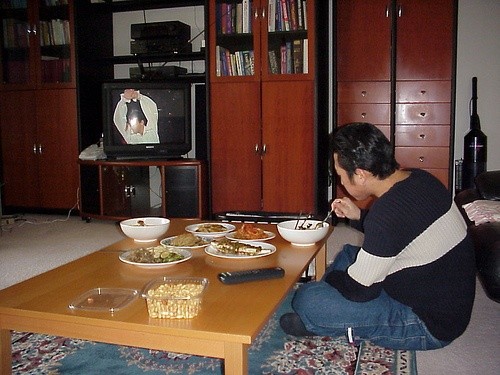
[8,264,418,375]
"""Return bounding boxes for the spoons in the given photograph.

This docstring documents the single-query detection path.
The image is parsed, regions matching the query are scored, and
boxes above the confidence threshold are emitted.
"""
[314,200,341,230]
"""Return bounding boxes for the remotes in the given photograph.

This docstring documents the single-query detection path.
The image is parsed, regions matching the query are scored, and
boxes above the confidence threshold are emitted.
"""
[217,267,285,283]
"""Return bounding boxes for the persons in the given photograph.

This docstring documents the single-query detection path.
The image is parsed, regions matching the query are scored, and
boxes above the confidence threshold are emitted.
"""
[279,122,477,351]
[114,89,159,144]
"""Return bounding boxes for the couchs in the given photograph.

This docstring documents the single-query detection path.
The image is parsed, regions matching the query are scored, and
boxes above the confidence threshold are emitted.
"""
[455,170,500,303]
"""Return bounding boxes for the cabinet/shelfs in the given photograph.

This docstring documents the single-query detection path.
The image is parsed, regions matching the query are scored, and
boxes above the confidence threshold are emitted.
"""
[0,0,459,223]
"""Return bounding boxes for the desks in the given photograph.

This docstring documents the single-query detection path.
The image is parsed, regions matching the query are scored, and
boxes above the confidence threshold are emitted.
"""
[0,218,333,375]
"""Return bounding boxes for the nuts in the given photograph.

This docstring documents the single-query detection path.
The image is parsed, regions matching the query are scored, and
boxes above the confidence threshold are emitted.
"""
[145,283,204,319]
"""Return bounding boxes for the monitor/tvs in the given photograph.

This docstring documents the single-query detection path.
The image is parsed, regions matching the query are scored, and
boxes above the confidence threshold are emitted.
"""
[102,82,193,162]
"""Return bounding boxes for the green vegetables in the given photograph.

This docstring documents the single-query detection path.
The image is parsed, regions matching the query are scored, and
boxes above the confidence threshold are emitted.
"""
[154,252,184,262]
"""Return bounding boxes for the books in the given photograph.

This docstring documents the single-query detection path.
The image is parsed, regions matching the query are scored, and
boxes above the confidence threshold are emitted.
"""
[268,39,308,74]
[268,0,307,32]
[215,46,254,76]
[0,0,71,83]
[216,0,252,33]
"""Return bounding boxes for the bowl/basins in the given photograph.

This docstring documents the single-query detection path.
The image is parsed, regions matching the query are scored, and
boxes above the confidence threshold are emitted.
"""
[276,219,330,245]
[140,275,210,319]
[120,217,170,242]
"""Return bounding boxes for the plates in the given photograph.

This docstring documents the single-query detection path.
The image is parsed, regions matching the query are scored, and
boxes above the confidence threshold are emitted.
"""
[204,241,277,259]
[118,245,192,267]
[185,223,235,235]
[225,231,276,241]
[161,234,219,250]
